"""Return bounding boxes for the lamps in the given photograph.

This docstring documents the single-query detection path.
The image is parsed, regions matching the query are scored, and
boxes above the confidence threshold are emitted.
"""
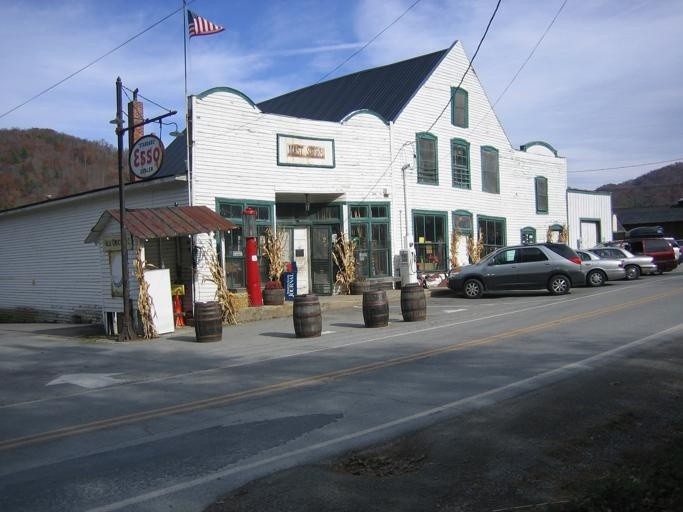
[305,194,311,212]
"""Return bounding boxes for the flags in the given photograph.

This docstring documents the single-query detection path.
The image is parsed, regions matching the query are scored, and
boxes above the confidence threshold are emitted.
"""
[187,8,226,40]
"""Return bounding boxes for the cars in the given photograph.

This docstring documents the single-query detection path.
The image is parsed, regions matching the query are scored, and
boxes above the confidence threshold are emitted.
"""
[448,223,681,300]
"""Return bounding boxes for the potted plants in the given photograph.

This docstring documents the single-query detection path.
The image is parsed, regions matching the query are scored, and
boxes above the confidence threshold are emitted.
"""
[262,273,286,305]
[350,276,370,295]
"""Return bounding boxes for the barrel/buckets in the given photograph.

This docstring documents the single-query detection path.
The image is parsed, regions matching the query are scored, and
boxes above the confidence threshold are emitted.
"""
[194,301,223,342]
[293,294,322,337]
[361,291,389,328]
[401,286,426,322]
[262,289,286,305]
